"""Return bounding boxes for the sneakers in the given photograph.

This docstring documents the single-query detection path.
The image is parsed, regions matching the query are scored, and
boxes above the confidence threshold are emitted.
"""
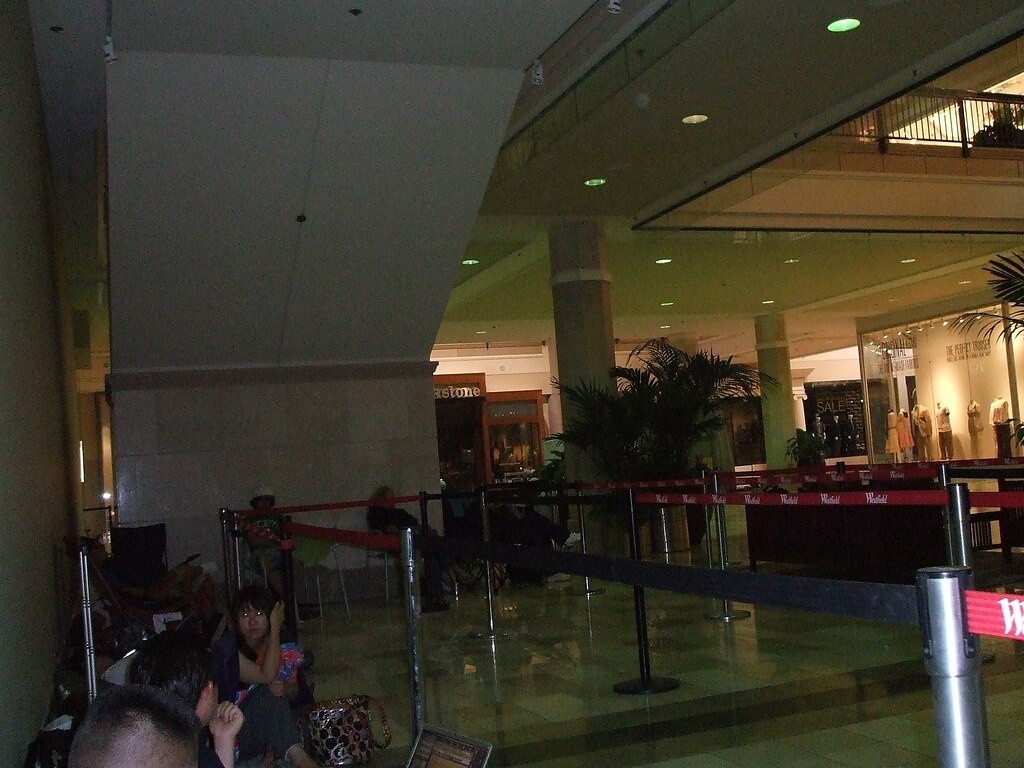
[545,572,570,582]
[565,530,582,547]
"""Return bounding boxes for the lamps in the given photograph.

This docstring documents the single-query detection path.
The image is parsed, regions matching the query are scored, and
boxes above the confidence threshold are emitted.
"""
[905,324,911,333]
[895,327,901,335]
[959,314,964,323]
[883,330,888,339]
[975,308,981,320]
[917,322,923,331]
[867,337,873,345]
[940,317,948,326]
[929,319,935,328]
[992,305,998,315]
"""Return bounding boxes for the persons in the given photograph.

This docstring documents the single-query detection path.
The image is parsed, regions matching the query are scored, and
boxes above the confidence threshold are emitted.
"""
[936,401,954,460]
[967,400,984,458]
[989,396,1012,459]
[884,409,900,453]
[895,408,915,448]
[366,486,475,614]
[811,411,860,459]
[68,563,319,768]
[911,403,933,462]
[468,501,581,586]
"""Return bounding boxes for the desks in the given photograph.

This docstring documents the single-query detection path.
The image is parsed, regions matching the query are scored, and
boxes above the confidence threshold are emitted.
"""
[746,483,951,576]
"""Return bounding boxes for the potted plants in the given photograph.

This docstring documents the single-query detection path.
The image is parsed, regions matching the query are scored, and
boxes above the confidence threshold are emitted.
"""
[537,336,785,557]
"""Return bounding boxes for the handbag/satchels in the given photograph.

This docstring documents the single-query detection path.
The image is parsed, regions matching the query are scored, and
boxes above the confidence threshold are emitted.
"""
[296,694,392,768]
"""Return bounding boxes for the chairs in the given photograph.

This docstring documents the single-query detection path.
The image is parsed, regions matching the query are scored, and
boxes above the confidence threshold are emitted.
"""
[364,528,527,610]
[242,515,354,630]
